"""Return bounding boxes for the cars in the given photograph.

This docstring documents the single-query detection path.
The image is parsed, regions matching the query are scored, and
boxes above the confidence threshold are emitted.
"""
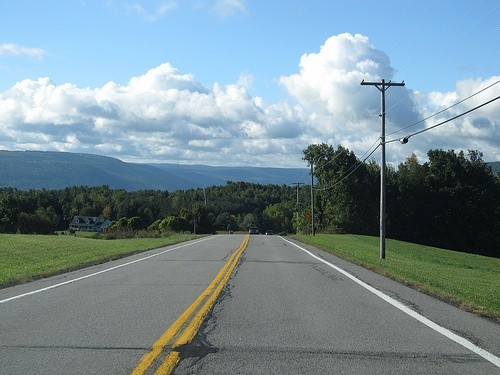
[248,227,259,234]
[265,230,273,235]
